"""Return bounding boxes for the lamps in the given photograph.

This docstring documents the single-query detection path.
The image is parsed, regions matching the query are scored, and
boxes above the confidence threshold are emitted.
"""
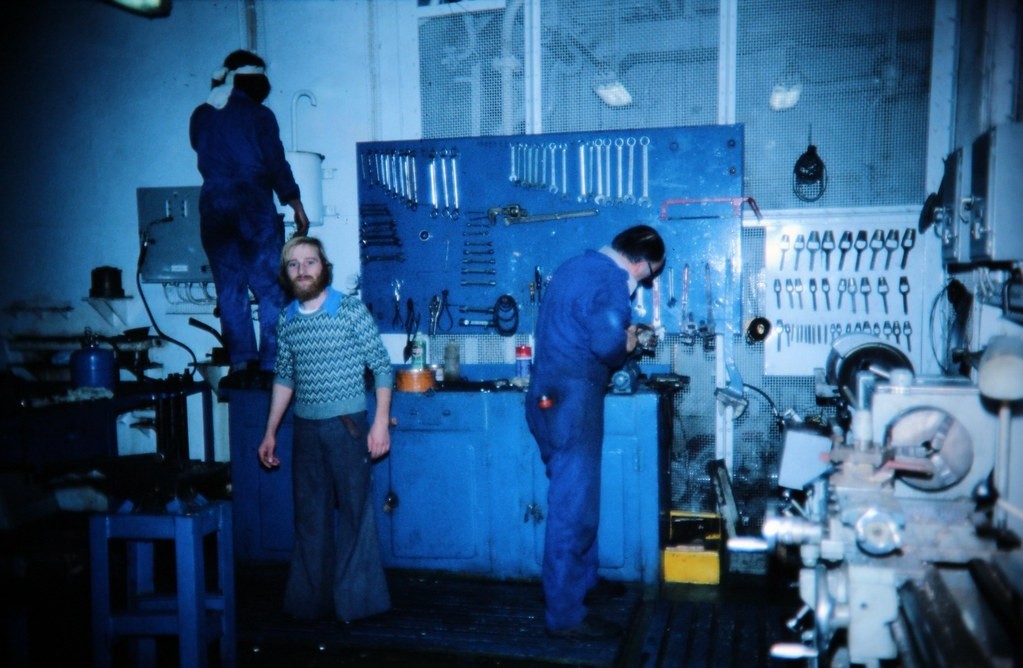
[772,80,802,113]
[591,72,632,108]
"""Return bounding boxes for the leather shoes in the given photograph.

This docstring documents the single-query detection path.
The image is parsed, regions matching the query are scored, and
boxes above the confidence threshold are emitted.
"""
[546,610,623,642]
[584,577,628,600]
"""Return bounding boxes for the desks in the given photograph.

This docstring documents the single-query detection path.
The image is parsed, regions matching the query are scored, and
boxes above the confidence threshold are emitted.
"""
[0,380,211,561]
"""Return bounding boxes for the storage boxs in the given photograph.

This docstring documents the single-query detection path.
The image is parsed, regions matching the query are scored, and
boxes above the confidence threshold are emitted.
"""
[662,510,725,584]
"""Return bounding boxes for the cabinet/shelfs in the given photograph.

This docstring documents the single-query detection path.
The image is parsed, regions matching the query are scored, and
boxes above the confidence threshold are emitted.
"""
[220,385,667,584]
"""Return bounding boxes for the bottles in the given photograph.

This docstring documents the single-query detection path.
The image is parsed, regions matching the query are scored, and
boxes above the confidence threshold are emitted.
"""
[444,339,459,380]
[515,344,532,392]
[412,332,426,370]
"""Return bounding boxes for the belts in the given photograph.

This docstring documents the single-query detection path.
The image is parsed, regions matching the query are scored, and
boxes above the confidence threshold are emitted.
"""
[340,414,361,441]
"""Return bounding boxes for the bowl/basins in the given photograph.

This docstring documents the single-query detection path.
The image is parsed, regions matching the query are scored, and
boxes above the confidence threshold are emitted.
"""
[123,326,150,340]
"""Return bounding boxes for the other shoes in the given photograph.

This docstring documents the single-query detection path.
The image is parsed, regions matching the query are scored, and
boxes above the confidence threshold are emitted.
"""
[218,361,276,392]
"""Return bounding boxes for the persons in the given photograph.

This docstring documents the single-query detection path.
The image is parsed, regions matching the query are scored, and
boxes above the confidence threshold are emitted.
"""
[526,225,665,627]
[189,51,310,387]
[257,236,394,620]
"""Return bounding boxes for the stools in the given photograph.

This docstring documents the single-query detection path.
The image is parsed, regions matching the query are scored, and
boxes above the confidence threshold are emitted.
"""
[89,499,236,667]
